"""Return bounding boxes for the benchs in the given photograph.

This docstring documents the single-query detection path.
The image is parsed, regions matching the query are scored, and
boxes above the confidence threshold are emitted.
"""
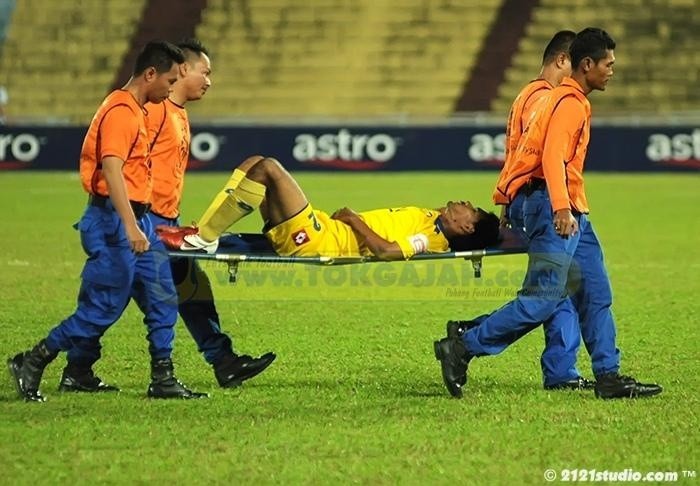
[0,0,699,122]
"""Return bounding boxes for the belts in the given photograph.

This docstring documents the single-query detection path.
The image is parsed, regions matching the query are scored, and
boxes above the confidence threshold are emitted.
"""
[84,192,155,222]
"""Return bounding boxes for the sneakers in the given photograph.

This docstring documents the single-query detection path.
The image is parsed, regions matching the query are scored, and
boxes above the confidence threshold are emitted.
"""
[155,223,220,255]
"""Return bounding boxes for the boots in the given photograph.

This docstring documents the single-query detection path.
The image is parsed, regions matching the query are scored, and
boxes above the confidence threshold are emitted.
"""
[146,356,210,401]
[432,335,477,401]
[6,337,55,404]
[55,359,122,393]
[540,340,599,391]
[209,350,279,391]
[445,317,483,338]
[593,355,666,401]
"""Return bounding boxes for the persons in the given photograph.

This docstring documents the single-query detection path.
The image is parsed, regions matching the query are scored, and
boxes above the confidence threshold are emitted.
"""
[56,38,276,395]
[7,39,211,406]
[154,154,501,264]
[447,31,600,393]
[434,26,664,400]
